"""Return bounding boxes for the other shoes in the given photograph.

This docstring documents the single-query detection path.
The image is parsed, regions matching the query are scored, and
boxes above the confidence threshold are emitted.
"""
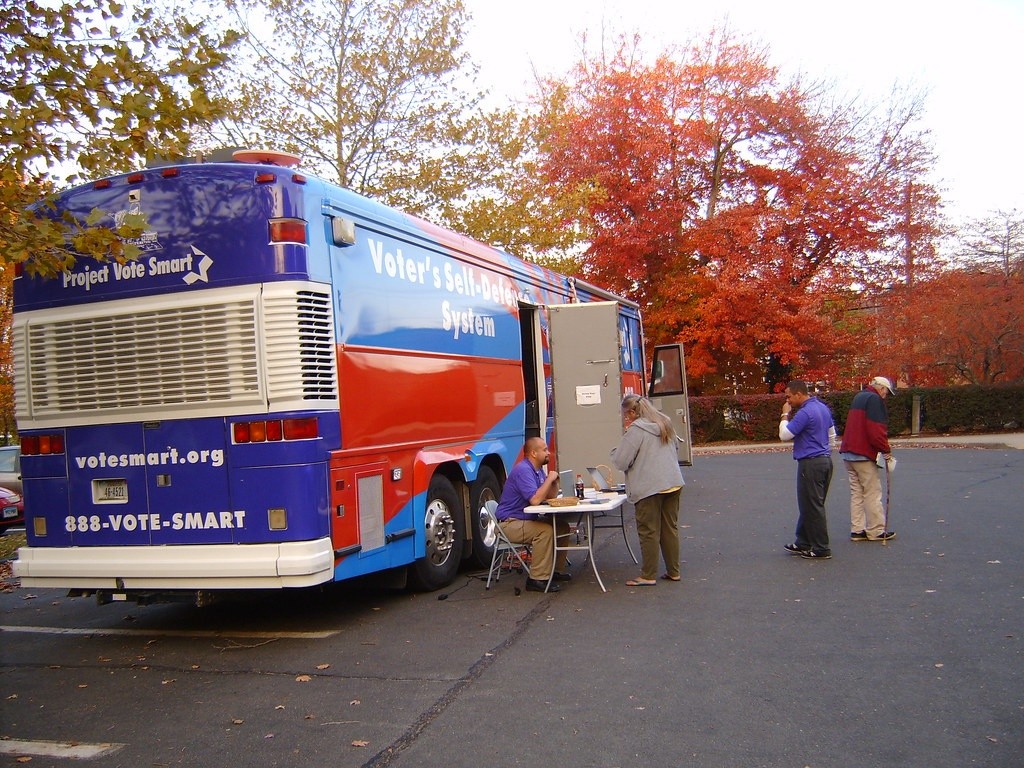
[801,549,832,558]
[850,529,869,541]
[869,531,896,540]
[784,542,809,554]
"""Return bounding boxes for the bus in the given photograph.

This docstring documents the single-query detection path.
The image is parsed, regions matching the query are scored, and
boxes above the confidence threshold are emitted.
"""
[9,148,698,607]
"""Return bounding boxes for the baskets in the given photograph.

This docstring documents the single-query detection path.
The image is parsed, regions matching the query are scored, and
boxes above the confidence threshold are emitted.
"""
[591,465,612,490]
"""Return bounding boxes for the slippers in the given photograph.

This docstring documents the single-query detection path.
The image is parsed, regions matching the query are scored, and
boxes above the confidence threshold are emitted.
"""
[665,571,680,581]
[625,578,657,585]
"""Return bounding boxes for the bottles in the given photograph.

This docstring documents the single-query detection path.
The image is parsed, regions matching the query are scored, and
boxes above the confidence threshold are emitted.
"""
[575,474,584,500]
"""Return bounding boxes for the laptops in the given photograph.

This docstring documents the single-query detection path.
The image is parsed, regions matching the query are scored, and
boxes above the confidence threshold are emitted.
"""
[539,470,575,505]
[586,468,626,494]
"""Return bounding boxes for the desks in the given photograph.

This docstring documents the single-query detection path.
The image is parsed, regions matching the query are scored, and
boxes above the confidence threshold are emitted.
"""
[524,487,639,593]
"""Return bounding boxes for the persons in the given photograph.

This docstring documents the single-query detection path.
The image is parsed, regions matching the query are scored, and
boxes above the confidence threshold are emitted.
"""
[778,380,837,559]
[492,436,571,593]
[610,394,686,586]
[838,377,896,542]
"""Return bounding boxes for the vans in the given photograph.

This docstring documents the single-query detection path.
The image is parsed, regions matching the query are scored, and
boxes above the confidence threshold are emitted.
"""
[0,446,26,497]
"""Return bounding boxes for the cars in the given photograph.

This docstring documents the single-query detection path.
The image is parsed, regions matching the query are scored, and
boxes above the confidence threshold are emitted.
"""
[0,487,24,535]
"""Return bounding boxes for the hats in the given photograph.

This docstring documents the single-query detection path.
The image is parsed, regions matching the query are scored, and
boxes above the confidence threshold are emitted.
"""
[872,376,896,396]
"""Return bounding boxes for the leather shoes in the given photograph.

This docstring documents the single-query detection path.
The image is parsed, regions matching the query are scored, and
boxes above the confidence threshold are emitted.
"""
[526,577,560,592]
[552,572,572,582]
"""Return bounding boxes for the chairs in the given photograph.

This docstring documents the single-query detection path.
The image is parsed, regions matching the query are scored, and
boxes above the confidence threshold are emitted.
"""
[485,500,532,590]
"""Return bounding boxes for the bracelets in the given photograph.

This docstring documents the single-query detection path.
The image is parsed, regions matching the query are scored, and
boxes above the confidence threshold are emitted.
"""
[780,413,789,418]
